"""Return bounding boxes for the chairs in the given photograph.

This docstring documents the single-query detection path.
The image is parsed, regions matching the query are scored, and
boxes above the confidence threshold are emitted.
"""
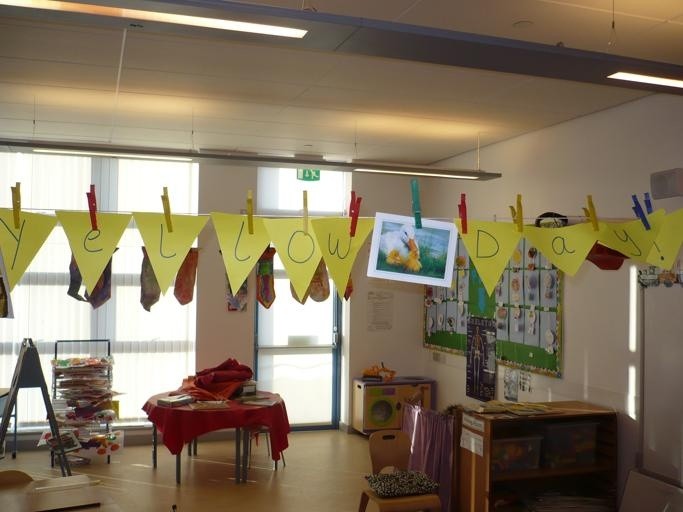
[359,430,442,512]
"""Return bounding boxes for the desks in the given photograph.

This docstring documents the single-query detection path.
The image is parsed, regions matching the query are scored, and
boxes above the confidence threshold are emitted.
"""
[145,385,286,484]
[0,388,18,460]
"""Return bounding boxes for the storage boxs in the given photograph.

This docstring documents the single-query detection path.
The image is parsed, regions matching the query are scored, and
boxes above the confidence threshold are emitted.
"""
[490,420,601,473]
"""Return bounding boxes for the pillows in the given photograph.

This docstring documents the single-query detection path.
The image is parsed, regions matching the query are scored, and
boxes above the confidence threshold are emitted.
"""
[364,470,441,498]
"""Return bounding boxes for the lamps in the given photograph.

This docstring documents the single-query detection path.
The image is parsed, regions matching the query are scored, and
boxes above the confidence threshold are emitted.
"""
[0,1,309,39]
[3,108,502,181]
[600,0,683,95]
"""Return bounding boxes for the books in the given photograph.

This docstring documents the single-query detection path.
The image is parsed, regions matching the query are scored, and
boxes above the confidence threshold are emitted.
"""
[361,375,384,383]
[187,402,232,411]
[242,399,277,406]
[157,394,191,408]
